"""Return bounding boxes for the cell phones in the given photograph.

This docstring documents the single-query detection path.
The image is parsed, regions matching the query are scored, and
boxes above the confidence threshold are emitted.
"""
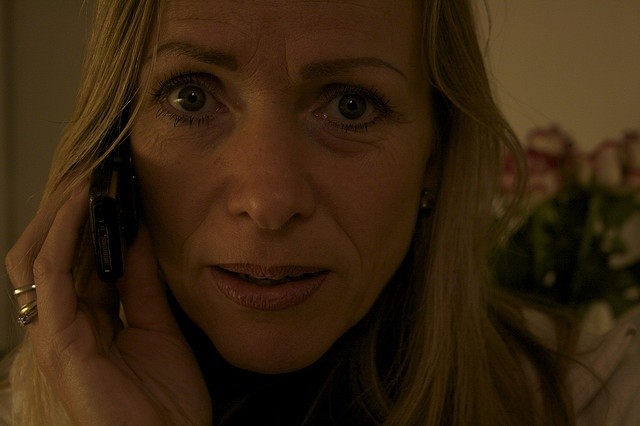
[86,143,128,283]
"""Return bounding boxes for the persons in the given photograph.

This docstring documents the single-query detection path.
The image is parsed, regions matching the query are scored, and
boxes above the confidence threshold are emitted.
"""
[0,1,613,426]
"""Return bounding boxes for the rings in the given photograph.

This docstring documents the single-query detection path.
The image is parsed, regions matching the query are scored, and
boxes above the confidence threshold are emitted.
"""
[16,300,39,327]
[12,283,36,296]
